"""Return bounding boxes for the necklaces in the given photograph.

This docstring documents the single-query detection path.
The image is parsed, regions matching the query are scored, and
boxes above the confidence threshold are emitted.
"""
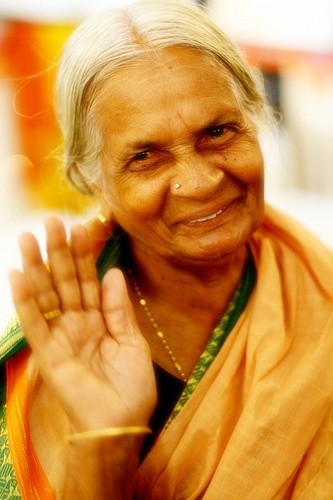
[127,266,189,384]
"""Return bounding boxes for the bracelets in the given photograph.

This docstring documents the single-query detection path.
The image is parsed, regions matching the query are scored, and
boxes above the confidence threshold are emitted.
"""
[68,424,152,444]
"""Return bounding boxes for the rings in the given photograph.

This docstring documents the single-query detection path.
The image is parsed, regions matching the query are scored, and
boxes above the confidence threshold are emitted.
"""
[43,309,62,321]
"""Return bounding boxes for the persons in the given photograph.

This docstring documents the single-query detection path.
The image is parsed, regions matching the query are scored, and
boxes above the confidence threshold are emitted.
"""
[0,0,333,500]
